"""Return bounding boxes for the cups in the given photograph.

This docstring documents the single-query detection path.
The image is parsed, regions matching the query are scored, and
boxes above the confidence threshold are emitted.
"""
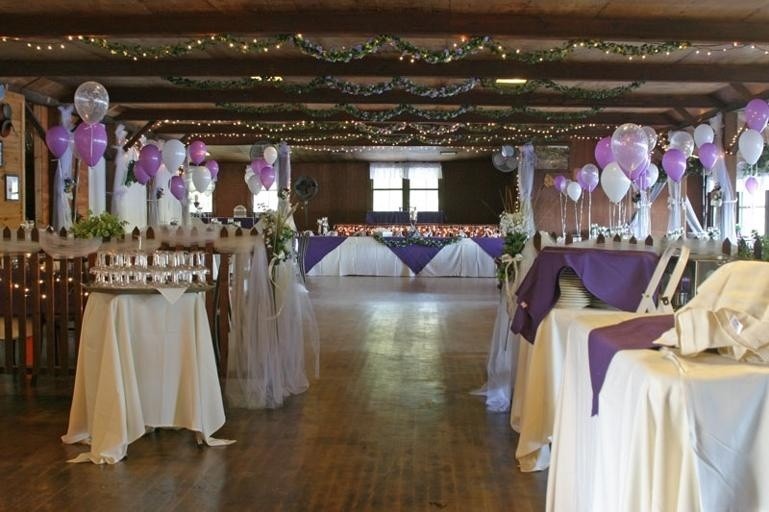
[668,290,688,307]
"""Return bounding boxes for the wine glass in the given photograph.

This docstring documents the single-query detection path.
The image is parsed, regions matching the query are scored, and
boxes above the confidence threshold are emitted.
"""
[95,249,207,290]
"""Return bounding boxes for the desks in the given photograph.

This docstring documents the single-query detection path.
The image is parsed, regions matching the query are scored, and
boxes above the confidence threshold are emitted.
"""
[511,312,606,473]
[545,311,769,512]
[60,292,239,464]
[296,236,503,277]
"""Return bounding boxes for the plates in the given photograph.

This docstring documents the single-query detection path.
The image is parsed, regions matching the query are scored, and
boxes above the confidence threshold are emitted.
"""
[555,273,620,310]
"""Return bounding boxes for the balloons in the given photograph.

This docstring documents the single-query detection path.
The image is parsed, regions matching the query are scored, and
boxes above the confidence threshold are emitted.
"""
[46,81,111,169]
[244,146,279,194]
[132,138,220,205]
[544,97,769,206]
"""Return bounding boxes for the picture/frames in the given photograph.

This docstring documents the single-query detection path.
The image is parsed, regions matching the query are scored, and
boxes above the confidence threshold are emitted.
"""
[5,175,20,201]
[0,141,3,167]
